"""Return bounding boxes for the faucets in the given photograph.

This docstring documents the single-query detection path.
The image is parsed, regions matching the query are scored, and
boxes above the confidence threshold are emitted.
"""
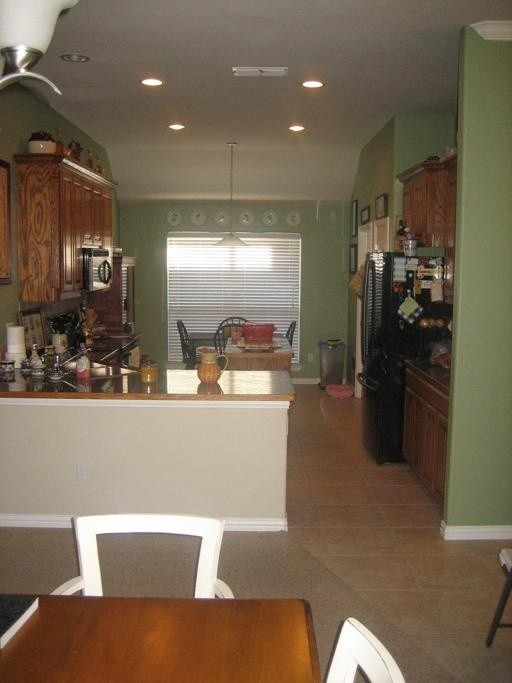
[52,346,92,368]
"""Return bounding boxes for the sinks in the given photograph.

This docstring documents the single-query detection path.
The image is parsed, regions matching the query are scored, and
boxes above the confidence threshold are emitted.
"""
[66,360,104,369]
[66,363,136,380]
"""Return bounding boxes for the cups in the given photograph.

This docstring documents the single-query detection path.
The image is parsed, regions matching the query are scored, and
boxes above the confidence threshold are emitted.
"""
[404,239,418,256]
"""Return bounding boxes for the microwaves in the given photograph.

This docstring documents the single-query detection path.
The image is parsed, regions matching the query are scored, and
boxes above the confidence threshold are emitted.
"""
[83,248,110,293]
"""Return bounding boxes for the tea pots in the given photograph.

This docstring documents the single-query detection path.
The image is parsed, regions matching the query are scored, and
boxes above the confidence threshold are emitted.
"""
[197,347,228,384]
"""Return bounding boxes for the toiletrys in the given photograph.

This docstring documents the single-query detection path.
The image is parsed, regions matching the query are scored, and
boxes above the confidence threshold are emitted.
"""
[29,344,41,359]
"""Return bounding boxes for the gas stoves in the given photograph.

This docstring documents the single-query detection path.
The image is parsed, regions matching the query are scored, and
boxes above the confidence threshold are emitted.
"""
[92,337,136,350]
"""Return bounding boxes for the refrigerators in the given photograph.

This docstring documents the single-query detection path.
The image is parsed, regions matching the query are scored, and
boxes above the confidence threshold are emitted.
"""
[355,251,428,465]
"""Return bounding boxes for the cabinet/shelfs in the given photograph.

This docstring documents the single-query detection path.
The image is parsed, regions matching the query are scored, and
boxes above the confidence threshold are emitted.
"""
[14,155,117,302]
[90,256,137,334]
[396,152,457,306]
[405,360,450,507]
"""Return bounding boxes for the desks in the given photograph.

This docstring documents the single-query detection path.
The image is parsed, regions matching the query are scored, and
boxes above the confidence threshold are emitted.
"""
[0,594,321,683]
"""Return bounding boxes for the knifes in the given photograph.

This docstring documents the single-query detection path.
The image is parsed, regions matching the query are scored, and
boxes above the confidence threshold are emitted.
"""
[71,318,85,332]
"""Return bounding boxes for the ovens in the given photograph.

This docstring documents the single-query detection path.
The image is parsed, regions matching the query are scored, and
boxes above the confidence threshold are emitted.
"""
[120,344,141,371]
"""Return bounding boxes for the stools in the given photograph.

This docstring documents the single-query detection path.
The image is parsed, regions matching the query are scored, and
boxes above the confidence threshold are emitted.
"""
[485,547,512,648]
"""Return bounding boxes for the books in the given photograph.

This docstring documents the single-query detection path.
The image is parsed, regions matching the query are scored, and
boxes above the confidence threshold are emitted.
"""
[0,594,40,649]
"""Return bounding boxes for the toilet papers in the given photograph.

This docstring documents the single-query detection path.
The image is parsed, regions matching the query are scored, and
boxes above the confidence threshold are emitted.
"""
[6,325,25,354]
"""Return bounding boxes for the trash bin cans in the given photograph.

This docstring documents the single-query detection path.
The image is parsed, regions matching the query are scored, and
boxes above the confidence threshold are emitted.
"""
[319,342,345,389]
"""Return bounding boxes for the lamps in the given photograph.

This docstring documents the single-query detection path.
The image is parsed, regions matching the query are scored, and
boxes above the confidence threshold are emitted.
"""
[0,0,80,96]
[211,142,252,247]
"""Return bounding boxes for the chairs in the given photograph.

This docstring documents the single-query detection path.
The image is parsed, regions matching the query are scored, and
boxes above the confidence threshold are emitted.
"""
[175,318,296,370]
[49,512,234,598]
[324,617,405,683]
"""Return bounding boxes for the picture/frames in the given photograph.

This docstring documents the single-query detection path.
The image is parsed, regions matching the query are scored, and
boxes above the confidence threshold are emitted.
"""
[350,193,388,277]
[18,307,47,357]
[0,160,12,285]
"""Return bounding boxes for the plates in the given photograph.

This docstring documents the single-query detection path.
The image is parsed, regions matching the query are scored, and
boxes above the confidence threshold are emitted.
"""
[244,346,268,350]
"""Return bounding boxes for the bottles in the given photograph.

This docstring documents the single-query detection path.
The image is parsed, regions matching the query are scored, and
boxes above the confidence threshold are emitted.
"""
[69,142,106,175]
[75,344,90,379]
